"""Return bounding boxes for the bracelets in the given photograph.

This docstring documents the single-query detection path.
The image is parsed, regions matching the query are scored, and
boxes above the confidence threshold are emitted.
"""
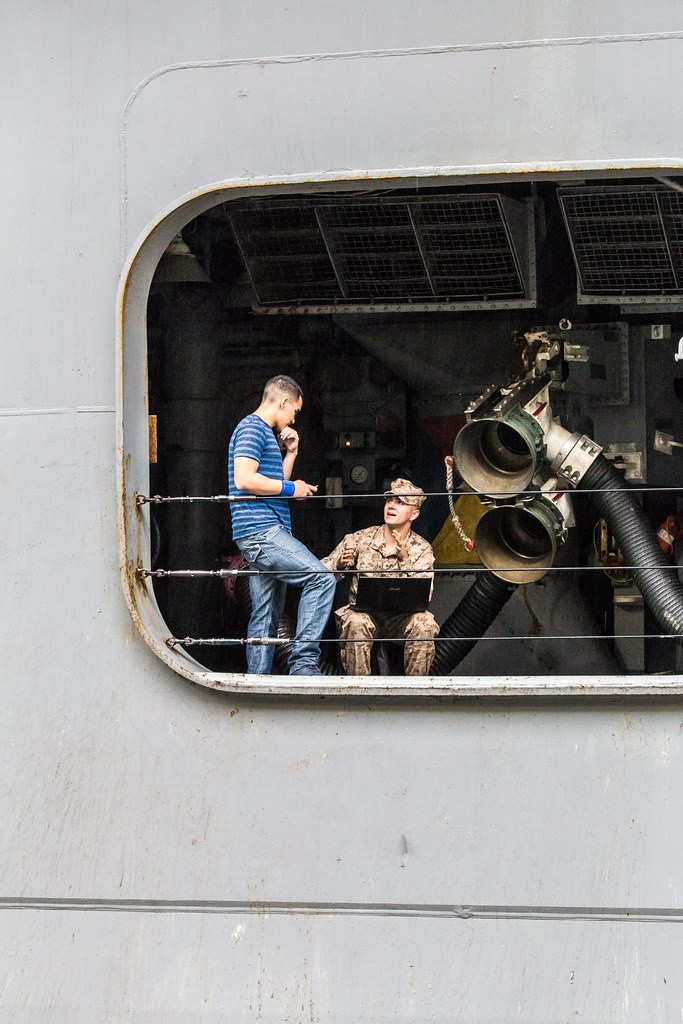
[279,480,295,496]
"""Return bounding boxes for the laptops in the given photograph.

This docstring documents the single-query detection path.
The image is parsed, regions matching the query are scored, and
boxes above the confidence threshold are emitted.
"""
[350,577,433,612]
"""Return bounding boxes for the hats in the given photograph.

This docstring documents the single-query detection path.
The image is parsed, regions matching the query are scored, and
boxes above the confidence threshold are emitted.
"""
[382,478,427,506]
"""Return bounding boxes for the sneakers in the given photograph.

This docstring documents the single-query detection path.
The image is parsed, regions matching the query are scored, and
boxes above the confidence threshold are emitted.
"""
[291,664,323,677]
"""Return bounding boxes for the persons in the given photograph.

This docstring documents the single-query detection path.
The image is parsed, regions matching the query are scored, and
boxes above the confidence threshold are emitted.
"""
[228,375,335,676]
[319,478,441,676]
